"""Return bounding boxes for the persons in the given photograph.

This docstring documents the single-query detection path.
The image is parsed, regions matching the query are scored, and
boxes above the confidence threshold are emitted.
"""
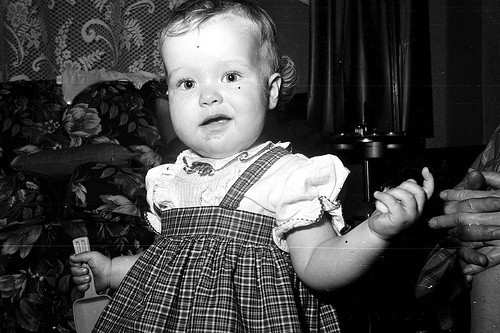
[425,123,500,332]
[69,1,436,332]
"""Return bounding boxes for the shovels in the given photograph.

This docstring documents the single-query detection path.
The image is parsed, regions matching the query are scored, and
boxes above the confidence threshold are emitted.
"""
[66,236,114,333]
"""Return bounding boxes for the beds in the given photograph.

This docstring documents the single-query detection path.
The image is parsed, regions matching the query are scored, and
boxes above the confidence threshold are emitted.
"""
[0,72,189,333]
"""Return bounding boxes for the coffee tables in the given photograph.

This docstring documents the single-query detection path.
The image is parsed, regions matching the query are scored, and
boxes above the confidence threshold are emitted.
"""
[319,132,408,221]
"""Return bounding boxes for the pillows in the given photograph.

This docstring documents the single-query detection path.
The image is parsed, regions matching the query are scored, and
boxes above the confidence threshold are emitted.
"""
[11,144,137,177]
[63,69,156,104]
